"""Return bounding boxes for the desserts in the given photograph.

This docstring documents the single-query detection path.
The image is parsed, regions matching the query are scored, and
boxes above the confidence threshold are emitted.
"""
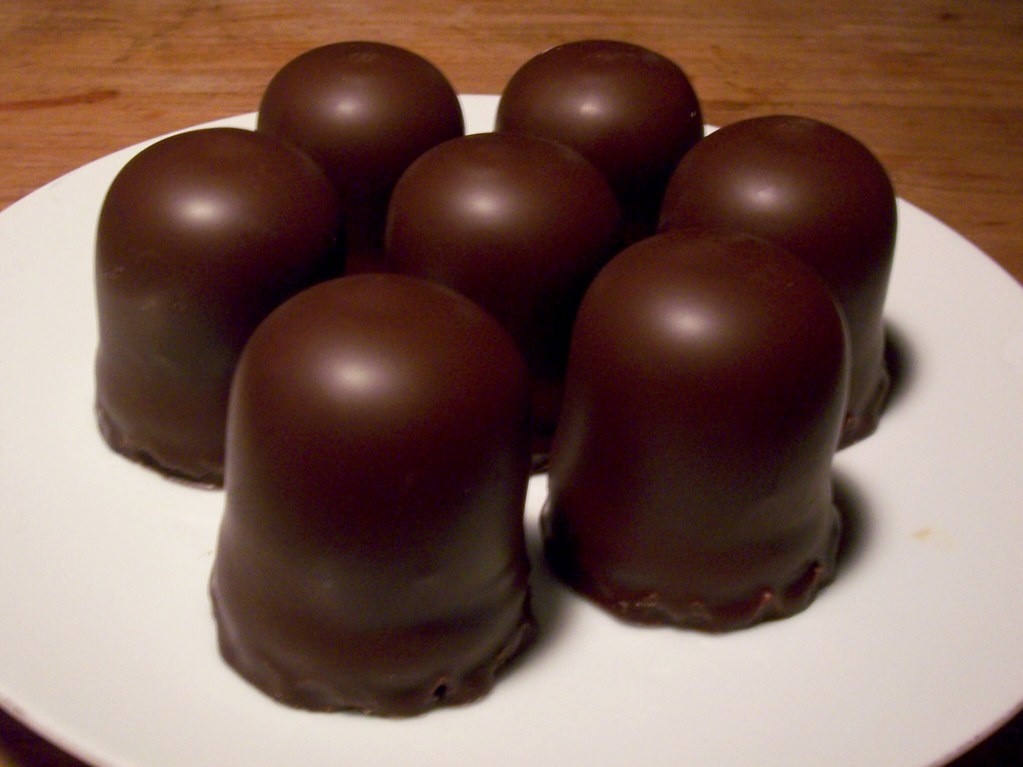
[93,39,898,718]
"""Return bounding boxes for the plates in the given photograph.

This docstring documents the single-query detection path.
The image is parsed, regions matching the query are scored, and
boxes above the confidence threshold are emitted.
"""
[1,94,1023,766]
